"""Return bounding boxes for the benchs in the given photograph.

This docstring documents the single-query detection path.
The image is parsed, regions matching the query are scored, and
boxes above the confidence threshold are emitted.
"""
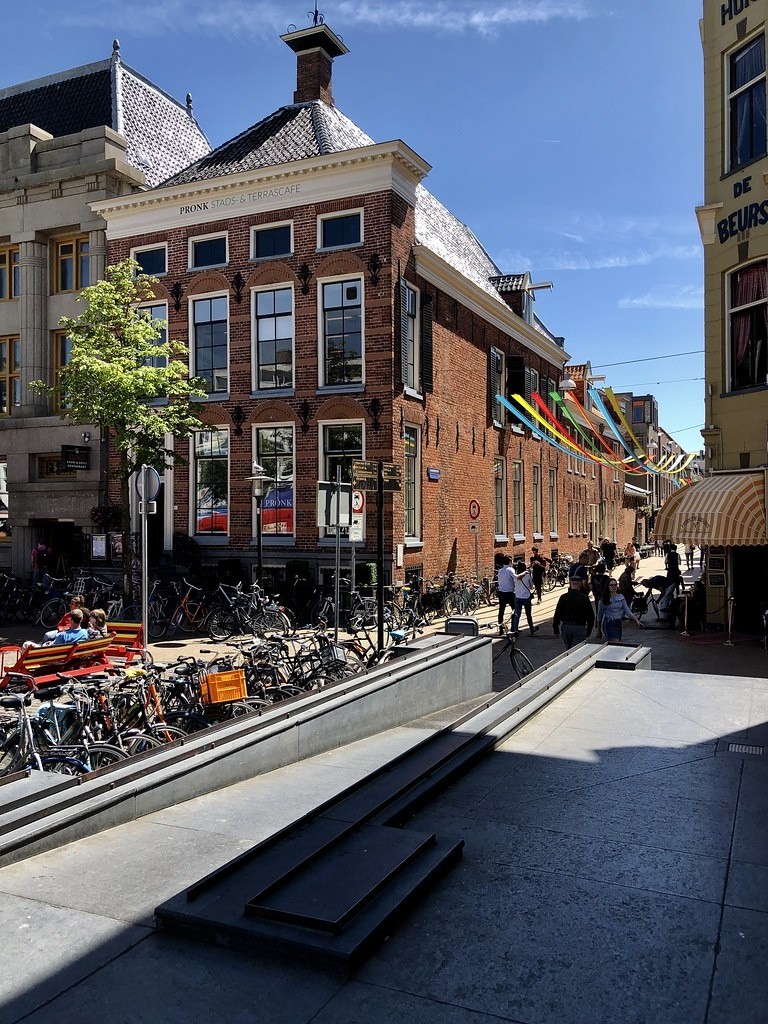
[0,622,149,692]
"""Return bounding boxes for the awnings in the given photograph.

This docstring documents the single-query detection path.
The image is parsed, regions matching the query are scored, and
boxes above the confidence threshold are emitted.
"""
[653,472,768,547]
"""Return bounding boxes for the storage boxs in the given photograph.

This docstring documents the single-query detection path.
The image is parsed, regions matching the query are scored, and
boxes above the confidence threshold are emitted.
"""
[200,668,249,704]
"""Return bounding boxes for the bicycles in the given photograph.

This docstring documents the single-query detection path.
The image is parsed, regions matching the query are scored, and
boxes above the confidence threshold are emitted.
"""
[0,557,501,642]
[479,609,536,680]
[0,609,425,779]
[627,579,659,623]
[544,551,570,593]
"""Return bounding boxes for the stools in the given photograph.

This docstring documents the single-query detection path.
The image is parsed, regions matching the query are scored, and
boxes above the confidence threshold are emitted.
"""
[0,646,22,678]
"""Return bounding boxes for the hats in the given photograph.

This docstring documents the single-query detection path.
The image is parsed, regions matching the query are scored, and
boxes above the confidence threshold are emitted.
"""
[571,576,581,581]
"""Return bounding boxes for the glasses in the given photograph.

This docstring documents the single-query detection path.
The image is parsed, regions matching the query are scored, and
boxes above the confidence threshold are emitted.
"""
[610,584,617,587]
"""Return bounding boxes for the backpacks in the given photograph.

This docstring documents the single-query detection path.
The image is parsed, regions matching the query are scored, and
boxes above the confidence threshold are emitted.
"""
[34,547,51,572]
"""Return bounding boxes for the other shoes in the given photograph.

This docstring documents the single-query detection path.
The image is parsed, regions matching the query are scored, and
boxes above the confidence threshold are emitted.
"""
[531,627,539,633]
[514,632,519,636]
[687,568,690,570]
[655,618,667,623]
[537,599,542,604]
[691,567,694,570]
[499,631,504,635]
[511,629,522,633]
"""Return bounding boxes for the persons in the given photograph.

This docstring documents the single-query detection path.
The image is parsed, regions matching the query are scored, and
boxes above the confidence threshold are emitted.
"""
[496,554,539,636]
[684,544,696,571]
[569,535,645,645]
[648,527,666,557]
[551,575,596,651]
[30,538,53,592]
[22,594,107,671]
[530,546,547,604]
[698,544,707,571]
[642,570,705,630]
[664,544,682,597]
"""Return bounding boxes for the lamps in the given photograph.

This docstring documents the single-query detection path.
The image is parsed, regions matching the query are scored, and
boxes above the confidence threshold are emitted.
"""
[298,398,313,433]
[171,282,183,311]
[231,404,246,434]
[367,397,384,427]
[297,262,314,295]
[368,252,383,287]
[232,271,246,304]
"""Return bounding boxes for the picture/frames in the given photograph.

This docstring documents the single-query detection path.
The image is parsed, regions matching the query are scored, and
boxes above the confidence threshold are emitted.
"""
[708,546,726,587]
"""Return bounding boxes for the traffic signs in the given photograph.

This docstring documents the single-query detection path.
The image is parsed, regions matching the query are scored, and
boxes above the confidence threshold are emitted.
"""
[350,458,403,493]
